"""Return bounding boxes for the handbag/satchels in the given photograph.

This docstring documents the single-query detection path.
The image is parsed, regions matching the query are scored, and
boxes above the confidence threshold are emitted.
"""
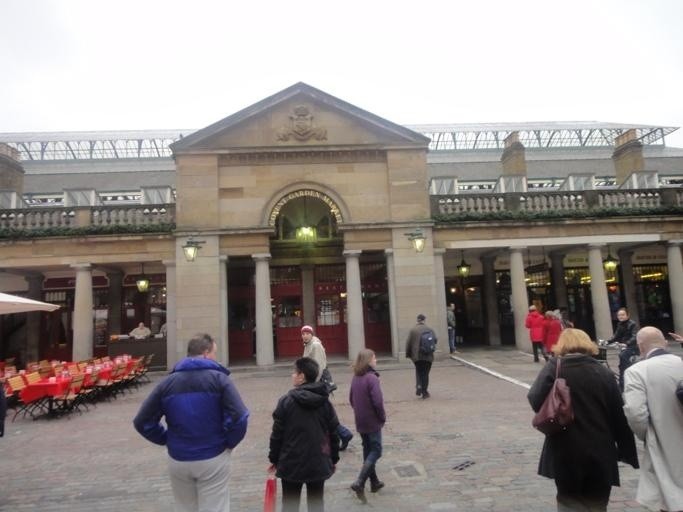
[420,330,435,354]
[532,378,576,438]
[264,478,277,512]
[320,370,336,394]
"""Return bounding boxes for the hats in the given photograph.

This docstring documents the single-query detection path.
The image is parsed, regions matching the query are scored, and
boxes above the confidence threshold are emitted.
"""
[301,325,313,333]
[417,314,426,321]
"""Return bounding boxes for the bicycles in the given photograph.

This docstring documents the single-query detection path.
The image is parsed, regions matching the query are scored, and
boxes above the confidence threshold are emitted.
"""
[592,338,640,383]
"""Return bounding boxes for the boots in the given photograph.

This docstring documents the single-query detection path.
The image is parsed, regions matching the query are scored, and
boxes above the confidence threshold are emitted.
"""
[369,465,384,491]
[351,462,373,502]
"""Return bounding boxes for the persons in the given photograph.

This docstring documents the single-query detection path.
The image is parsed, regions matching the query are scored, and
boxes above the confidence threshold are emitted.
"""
[405,313,438,399]
[266,357,341,512]
[588,313,597,340]
[528,330,640,511]
[605,307,639,390]
[128,322,152,338]
[623,327,683,511]
[300,325,353,451]
[446,301,468,354]
[133,333,250,511]
[525,304,574,363]
[252,319,256,358]
[348,348,387,505]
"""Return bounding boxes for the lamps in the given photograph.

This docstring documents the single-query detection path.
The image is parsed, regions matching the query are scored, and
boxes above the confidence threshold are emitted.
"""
[136,263,152,292]
[181,240,206,262]
[295,196,317,242]
[405,227,426,253]
[456,249,471,279]
[603,246,618,271]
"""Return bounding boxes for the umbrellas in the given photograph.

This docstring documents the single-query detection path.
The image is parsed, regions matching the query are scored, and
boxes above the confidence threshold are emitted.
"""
[0,293,63,316]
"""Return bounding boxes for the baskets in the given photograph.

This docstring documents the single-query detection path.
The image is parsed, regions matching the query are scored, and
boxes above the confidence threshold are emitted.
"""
[591,347,607,360]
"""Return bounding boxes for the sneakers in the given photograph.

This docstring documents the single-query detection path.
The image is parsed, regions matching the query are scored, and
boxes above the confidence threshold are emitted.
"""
[340,434,353,450]
[416,387,430,398]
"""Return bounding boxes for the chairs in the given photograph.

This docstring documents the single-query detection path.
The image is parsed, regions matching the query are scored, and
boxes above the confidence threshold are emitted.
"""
[0,354,155,423]
